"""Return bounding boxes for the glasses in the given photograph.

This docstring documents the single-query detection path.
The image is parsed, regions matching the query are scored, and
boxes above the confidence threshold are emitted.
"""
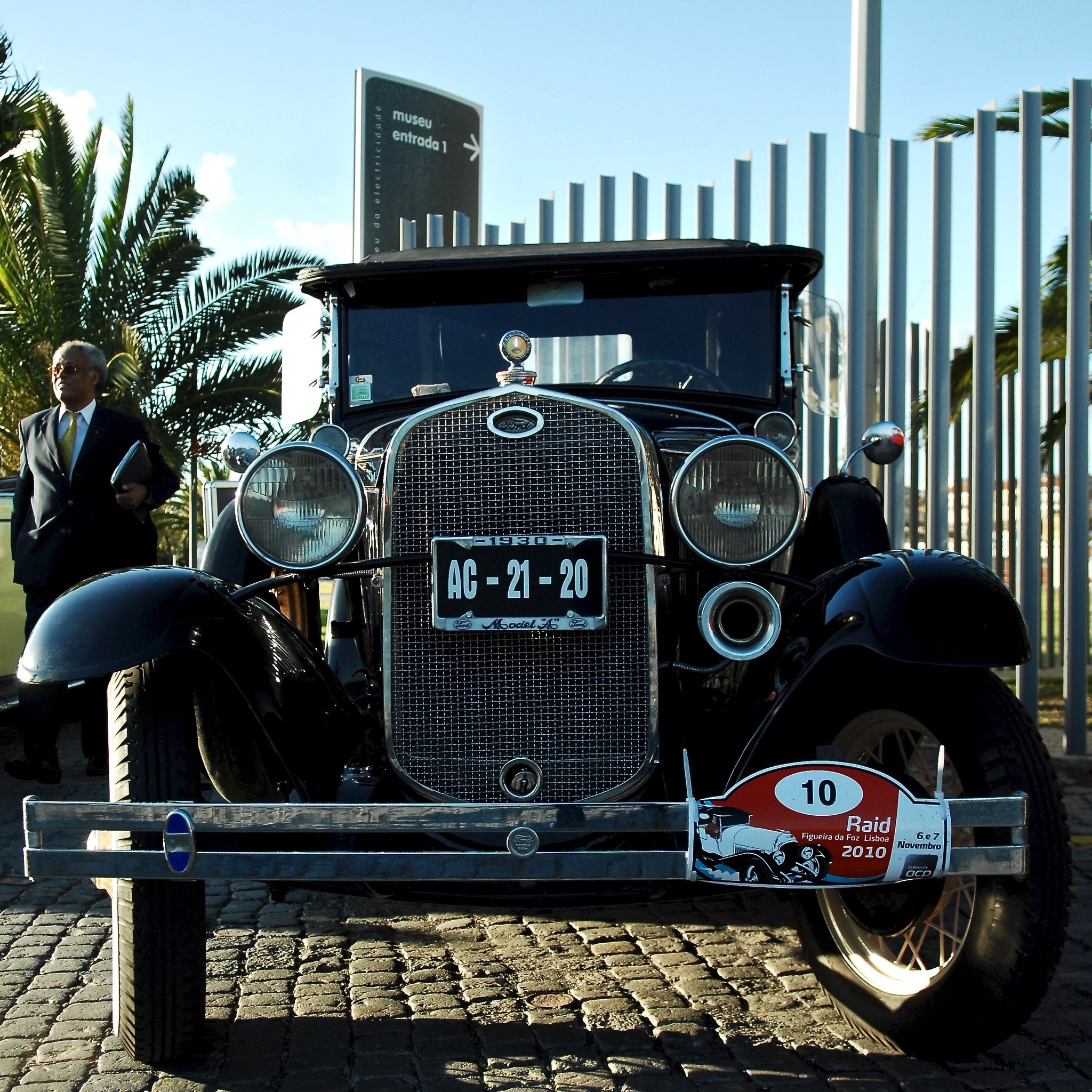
[47,363,91,377]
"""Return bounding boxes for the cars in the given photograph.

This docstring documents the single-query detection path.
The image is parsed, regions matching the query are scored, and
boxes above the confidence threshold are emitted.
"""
[12,237,1075,1065]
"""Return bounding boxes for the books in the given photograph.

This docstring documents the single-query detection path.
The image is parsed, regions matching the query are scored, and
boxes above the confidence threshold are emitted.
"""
[109,440,152,525]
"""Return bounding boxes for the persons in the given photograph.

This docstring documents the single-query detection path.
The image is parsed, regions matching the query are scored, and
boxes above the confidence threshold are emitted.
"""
[3,338,182,786]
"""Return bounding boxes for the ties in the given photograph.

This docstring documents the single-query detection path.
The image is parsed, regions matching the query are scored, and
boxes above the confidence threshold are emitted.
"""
[58,412,78,475]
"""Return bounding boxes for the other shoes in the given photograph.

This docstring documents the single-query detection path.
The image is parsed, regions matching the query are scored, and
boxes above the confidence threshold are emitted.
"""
[86,754,108,776]
[6,758,62,785]
